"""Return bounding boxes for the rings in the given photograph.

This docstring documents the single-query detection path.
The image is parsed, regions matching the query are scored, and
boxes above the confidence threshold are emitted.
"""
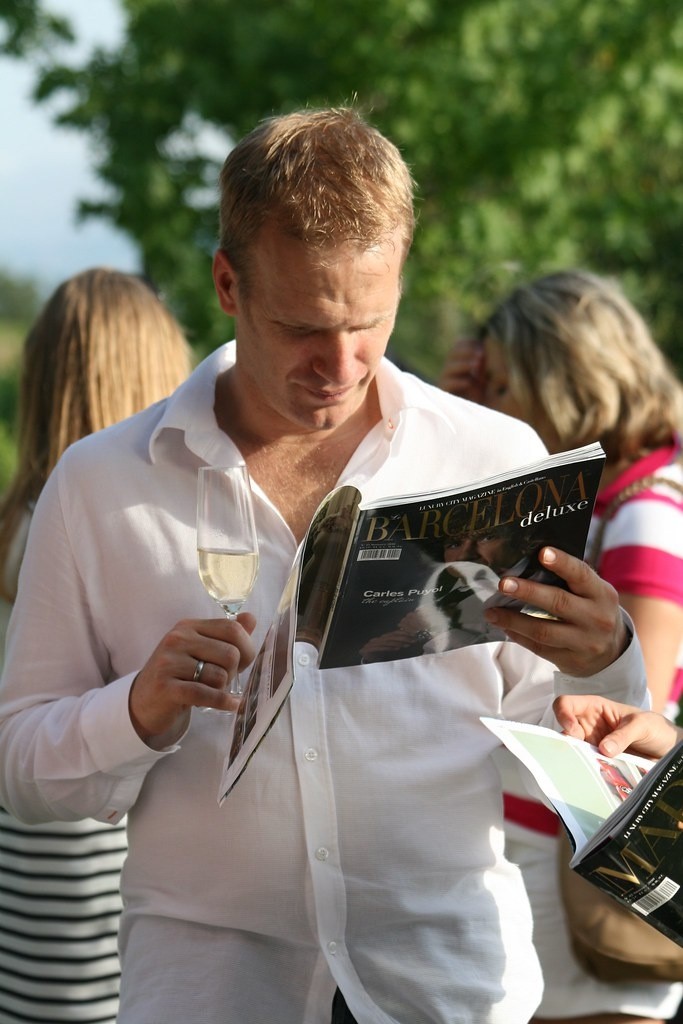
[192,660,205,682]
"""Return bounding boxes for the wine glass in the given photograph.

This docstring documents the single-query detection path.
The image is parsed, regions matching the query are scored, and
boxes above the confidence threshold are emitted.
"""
[195,463,260,716]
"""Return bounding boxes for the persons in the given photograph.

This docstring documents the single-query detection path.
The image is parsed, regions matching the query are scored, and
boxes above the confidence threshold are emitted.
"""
[1,105,683,1024]
[358,502,567,669]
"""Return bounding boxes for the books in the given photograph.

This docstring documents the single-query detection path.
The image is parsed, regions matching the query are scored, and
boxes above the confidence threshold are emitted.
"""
[479,716,683,949]
[214,440,608,809]
